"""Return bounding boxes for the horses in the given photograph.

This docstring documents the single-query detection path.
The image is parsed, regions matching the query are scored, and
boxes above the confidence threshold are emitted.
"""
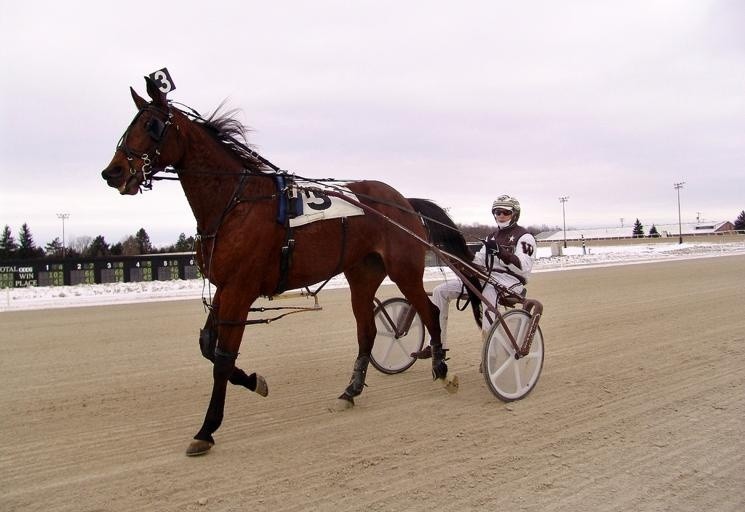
[101,76,484,456]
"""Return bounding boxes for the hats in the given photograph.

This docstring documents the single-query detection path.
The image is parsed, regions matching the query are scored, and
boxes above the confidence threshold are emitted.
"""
[493,205,514,211]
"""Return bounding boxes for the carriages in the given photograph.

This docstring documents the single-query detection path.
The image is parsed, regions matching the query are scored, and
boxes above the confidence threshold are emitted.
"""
[99,75,547,458]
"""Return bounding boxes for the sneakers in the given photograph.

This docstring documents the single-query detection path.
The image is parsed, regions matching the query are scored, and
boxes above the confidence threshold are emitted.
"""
[410,346,447,358]
[479,356,495,373]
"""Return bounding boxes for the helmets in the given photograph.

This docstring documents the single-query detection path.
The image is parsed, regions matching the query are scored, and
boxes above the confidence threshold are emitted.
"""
[492,196,521,223]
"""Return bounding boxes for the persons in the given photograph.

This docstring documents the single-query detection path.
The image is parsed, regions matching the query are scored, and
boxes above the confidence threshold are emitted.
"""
[408,192,536,376]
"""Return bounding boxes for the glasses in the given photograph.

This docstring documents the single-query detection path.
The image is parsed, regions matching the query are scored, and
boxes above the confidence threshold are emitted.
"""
[495,209,512,216]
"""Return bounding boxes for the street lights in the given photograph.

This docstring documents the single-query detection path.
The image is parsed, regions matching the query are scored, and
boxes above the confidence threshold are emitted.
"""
[673,182,685,245]
[557,196,568,248]
[54,213,70,256]
